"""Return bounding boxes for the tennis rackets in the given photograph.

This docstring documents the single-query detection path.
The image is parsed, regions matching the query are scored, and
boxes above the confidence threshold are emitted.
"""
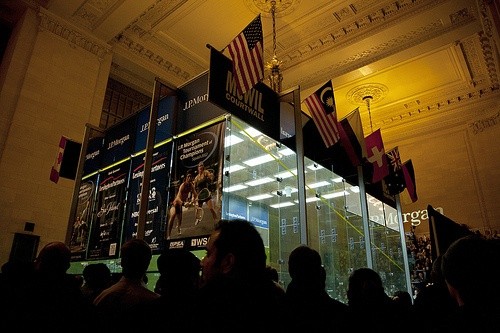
[194,203,204,221]
[185,188,211,208]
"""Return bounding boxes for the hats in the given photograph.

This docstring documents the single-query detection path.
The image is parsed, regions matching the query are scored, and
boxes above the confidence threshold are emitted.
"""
[187,169,193,174]
[198,161,204,166]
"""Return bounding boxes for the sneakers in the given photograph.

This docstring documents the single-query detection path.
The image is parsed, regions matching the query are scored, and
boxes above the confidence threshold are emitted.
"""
[80,245,85,250]
[176,226,182,235]
[195,218,201,226]
[167,236,171,239]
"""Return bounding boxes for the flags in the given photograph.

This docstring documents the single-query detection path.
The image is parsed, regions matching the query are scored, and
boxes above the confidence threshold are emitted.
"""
[403,160,418,204]
[304,80,341,149]
[382,147,407,197]
[362,129,389,184]
[50,136,69,183]
[227,14,264,96]
[339,109,369,167]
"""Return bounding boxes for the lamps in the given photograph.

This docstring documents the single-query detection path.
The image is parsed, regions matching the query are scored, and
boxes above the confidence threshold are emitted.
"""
[262,1,283,95]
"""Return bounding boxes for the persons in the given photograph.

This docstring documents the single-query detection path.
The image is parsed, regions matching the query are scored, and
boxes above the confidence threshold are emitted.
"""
[0,234,203,333]
[192,161,218,225]
[167,171,199,240]
[198,220,286,333]
[262,235,500,333]
